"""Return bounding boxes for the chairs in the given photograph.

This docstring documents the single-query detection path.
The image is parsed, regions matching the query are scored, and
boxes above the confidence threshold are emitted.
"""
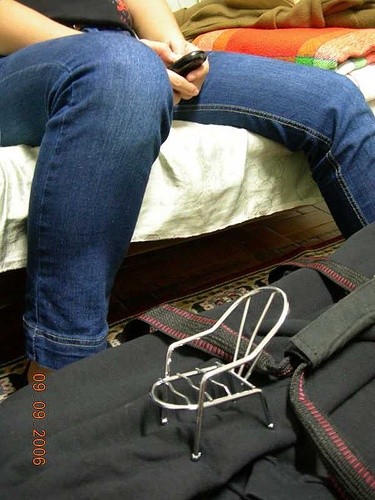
[147,284,291,461]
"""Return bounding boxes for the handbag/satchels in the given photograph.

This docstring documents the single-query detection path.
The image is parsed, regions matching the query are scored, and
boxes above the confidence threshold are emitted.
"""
[0,221,375,499]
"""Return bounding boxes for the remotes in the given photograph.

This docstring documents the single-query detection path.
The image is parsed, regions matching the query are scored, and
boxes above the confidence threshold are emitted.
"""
[167,50,207,76]
